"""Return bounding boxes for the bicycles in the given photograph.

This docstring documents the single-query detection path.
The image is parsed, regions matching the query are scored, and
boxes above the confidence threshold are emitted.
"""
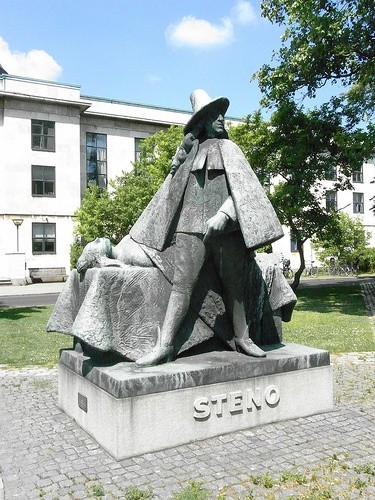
[275,251,357,279]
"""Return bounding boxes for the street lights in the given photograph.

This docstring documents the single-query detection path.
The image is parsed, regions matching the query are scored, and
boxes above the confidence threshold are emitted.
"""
[12,217,24,253]
